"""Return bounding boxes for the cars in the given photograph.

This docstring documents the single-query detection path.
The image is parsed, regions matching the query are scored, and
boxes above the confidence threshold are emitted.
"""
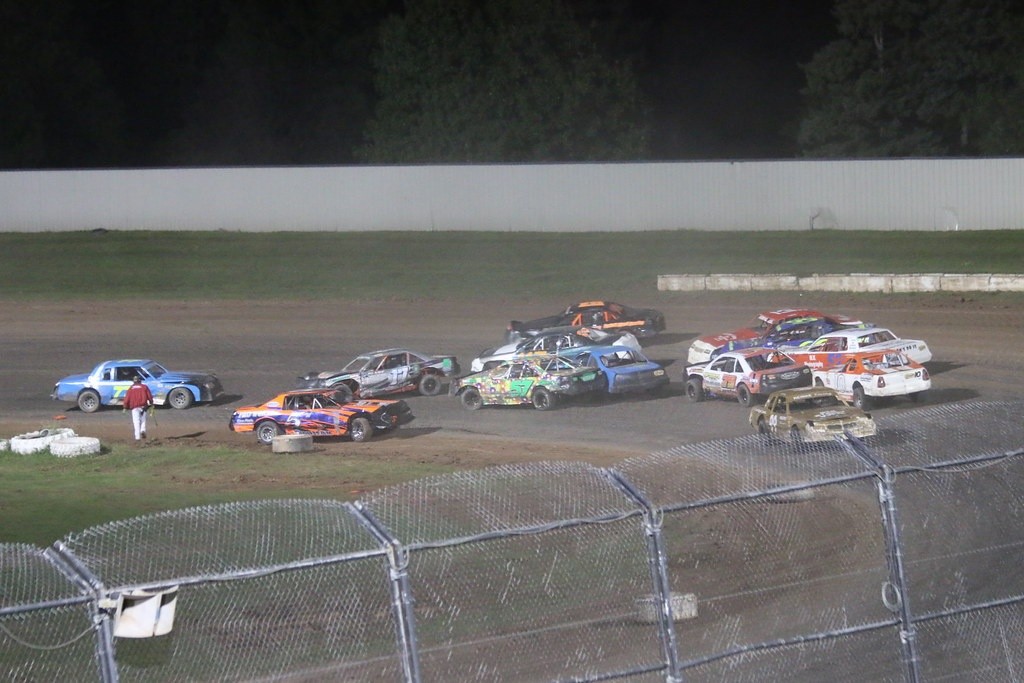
[687,305,877,365]
[471,324,642,372]
[709,316,887,364]
[453,354,607,411]
[228,388,416,445]
[297,348,461,401]
[503,301,667,345]
[810,349,932,411]
[540,344,671,394]
[681,346,812,407]
[749,385,877,454]
[779,327,933,371]
[50,359,225,414]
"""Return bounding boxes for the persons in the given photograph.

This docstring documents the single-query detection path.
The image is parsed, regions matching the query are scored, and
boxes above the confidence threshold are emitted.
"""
[122,375,154,444]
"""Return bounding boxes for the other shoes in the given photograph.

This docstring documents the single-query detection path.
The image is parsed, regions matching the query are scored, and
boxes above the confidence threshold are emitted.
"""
[141,431,146,438]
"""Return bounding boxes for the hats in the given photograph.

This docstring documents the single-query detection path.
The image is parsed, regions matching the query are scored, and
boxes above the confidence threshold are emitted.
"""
[134,376,140,382]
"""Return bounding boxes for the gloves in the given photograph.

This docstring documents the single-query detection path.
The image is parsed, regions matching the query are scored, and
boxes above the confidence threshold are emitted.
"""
[123,409,127,413]
